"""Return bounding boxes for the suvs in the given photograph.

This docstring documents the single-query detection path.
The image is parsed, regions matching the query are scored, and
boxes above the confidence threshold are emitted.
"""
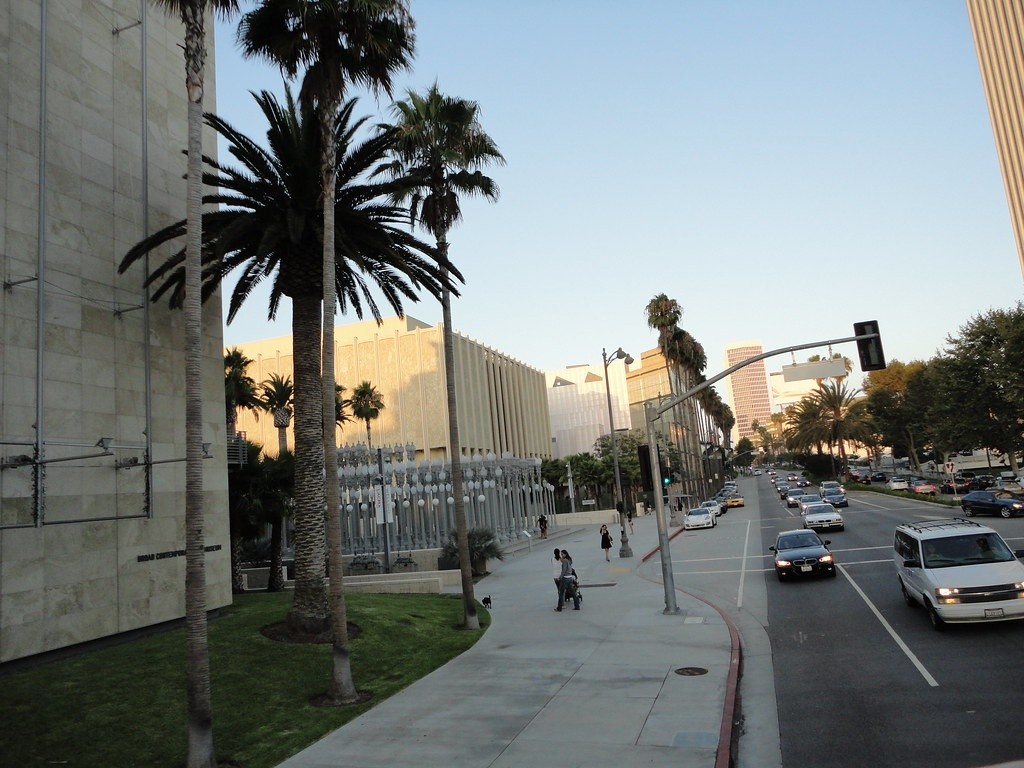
[961,488,1024,518]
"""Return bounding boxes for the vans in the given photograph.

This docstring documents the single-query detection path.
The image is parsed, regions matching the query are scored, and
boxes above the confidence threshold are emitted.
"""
[894,517,1024,632]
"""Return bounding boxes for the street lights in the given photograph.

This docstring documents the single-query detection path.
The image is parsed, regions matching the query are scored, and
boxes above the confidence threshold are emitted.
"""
[602,347,635,557]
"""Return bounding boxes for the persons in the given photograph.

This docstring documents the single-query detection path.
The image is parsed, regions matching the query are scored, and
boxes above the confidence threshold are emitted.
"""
[600,525,612,562]
[538,514,548,539]
[627,510,633,534]
[805,537,816,545]
[928,463,935,476]
[678,502,683,511]
[646,500,651,515]
[784,541,789,548]
[976,540,996,554]
[551,549,566,608]
[925,543,943,558]
[554,550,580,612]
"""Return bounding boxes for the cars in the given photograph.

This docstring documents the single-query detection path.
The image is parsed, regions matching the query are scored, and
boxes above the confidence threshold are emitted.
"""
[787,472,811,487]
[684,507,717,531]
[842,466,1024,496]
[769,529,837,582]
[786,489,807,508]
[819,480,849,508]
[699,480,744,517]
[753,466,793,500]
[797,495,844,533]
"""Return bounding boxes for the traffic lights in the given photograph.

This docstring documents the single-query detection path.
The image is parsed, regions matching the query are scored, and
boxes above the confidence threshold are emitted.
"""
[663,467,675,485]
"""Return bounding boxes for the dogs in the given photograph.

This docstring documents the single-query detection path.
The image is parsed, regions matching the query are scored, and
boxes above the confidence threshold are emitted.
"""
[482,595,491,609]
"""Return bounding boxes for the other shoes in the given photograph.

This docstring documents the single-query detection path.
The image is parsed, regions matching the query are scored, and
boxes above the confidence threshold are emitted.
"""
[554,608,562,612]
[572,607,580,610]
